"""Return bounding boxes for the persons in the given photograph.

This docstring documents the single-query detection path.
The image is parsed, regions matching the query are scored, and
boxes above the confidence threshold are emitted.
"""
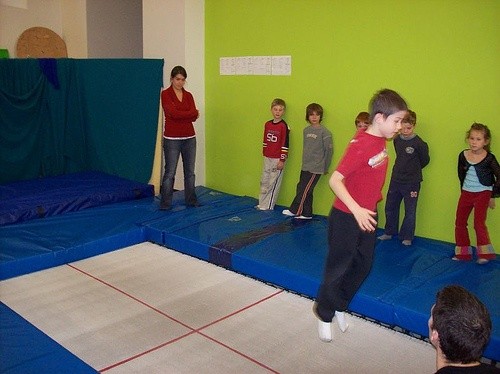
[256,99,289,211]
[282,103,334,219]
[376,109,431,245]
[160,66,201,209]
[427,285,500,374]
[354,112,370,133]
[312,89,408,342]
[452,123,500,264]
